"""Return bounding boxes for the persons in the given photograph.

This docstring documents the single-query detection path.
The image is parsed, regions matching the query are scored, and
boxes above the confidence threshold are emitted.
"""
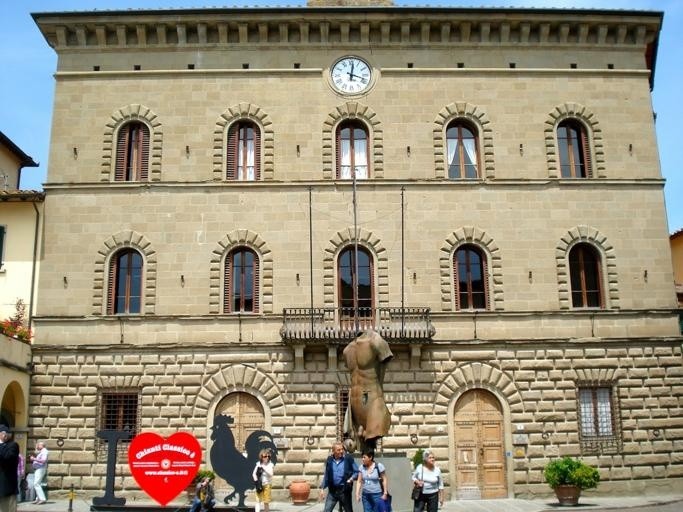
[189,478,215,512]
[30,442,48,505]
[355,450,393,512]
[0,423,19,512]
[254,450,274,512]
[16,444,25,503]
[319,444,358,511]
[0,425,9,445]
[412,450,445,512]
[342,332,393,443]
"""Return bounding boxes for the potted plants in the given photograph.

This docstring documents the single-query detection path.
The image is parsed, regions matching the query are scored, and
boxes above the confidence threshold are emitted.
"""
[544,456,601,507]
[186,469,214,504]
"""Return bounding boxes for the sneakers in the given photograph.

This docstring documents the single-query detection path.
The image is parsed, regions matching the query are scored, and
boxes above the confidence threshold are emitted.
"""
[32,500,46,504]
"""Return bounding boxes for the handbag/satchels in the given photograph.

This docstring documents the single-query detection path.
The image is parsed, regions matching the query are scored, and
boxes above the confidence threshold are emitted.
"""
[379,492,393,512]
[20,479,27,490]
[411,485,423,500]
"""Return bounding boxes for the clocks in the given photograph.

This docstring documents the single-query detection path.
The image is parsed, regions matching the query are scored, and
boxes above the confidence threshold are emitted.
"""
[330,56,372,95]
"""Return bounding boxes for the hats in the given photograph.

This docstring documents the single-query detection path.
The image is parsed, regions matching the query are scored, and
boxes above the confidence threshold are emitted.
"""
[0,425,8,432]
